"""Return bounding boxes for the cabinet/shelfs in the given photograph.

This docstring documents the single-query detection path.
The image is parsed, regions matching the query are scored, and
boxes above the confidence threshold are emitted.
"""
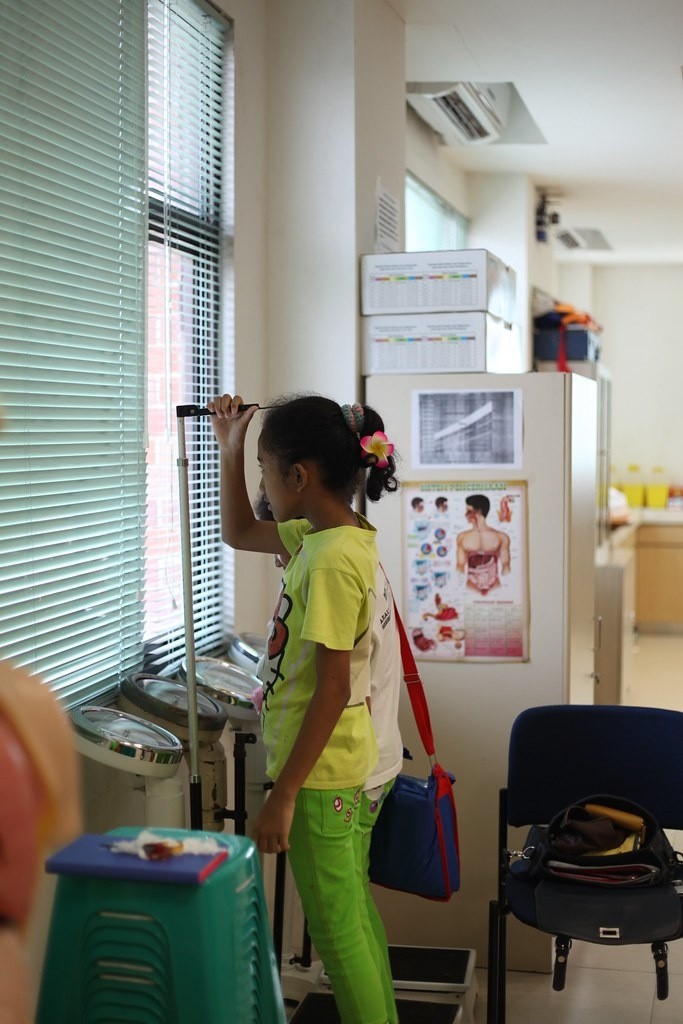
[605,507,683,634]
[593,550,638,704]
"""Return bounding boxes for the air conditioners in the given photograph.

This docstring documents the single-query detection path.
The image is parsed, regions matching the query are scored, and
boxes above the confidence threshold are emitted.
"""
[405,79,512,146]
[554,225,589,252]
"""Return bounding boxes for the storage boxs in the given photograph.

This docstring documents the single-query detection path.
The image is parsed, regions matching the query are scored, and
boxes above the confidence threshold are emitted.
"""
[357,313,520,374]
[533,323,610,382]
[357,250,517,321]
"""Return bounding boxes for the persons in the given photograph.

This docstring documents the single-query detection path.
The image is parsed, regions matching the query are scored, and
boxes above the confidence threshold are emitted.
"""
[207,394,403,1024]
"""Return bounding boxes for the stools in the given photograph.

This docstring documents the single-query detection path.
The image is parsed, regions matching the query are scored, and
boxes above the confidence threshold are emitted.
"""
[34,826,289,1024]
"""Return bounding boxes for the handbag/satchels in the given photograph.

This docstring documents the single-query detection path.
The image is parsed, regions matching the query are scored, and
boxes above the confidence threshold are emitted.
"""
[368,766,461,902]
[496,794,683,1000]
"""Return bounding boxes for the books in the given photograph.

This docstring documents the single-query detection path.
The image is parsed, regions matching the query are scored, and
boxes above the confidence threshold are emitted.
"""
[44,833,228,887]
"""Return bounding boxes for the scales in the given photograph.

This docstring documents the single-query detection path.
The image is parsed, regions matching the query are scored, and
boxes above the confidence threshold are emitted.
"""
[69,630,481,1024]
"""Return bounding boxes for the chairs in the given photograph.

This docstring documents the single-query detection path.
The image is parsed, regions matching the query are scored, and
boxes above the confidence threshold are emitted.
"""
[487,704,682,1024]
